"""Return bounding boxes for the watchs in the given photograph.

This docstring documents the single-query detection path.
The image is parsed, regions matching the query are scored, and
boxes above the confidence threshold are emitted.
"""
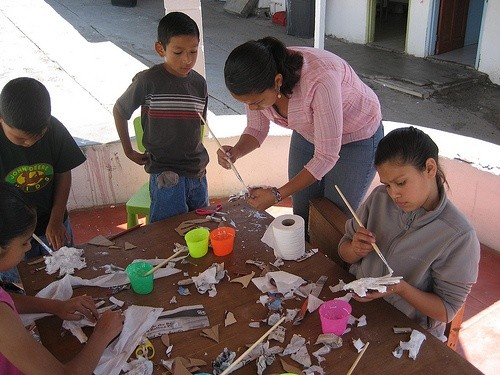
[272,188,281,203]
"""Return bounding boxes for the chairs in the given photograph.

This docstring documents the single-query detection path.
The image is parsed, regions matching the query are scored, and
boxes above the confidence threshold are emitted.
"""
[125,115,205,229]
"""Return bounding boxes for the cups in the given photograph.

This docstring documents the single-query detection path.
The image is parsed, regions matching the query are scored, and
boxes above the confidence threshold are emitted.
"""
[318,300,352,336]
[126,261,154,295]
[210,226,235,256]
[185,228,210,258]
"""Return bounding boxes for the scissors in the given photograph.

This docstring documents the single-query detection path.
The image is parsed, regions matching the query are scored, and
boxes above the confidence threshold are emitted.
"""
[135,338,155,360]
[196,204,228,220]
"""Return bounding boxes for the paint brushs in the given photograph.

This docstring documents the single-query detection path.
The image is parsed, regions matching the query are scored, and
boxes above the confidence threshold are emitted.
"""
[334,183,396,272]
[347,342,369,374]
[218,317,285,375]
[32,234,58,259]
[141,249,187,276]
[198,112,249,192]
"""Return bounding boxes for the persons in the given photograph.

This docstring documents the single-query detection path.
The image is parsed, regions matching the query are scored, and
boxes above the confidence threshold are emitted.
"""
[217,37,383,242]
[338,126,480,342]
[0,77,87,282]
[0,177,125,375]
[113,12,210,222]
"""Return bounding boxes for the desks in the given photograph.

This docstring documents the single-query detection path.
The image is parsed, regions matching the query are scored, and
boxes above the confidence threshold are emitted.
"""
[15,193,487,375]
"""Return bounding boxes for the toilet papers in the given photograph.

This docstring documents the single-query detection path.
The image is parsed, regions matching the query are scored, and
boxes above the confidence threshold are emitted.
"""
[260,214,306,261]
